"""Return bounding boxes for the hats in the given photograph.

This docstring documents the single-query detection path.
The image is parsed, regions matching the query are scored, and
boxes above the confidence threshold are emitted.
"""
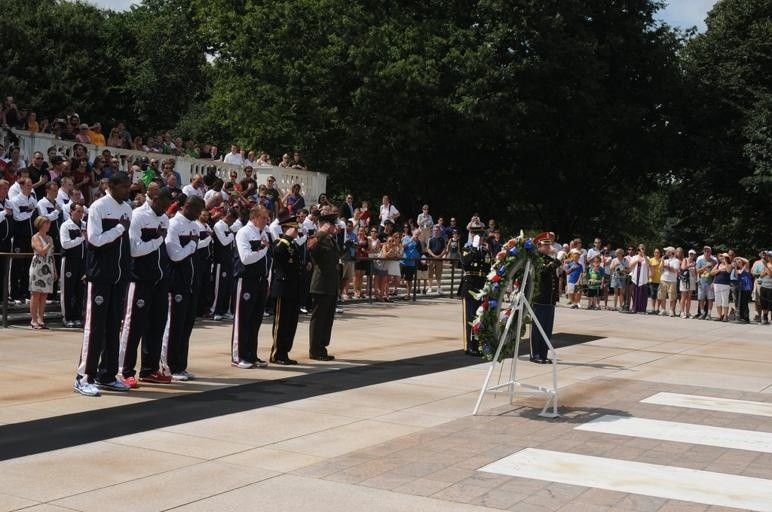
[663,246,712,254]
[278,216,300,229]
[533,232,552,245]
[470,226,488,237]
[318,213,339,224]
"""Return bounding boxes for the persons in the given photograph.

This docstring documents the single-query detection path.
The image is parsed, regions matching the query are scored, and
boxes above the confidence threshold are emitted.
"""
[0,96,307,170]
[118,190,173,387]
[547,233,772,326]
[426,224,448,295]
[328,192,504,301]
[1,137,328,320]
[530,230,567,364]
[72,170,132,396]
[160,196,205,383]
[271,214,308,366]
[455,228,493,357]
[307,214,343,361]
[27,215,59,331]
[231,205,270,370]
[59,201,88,328]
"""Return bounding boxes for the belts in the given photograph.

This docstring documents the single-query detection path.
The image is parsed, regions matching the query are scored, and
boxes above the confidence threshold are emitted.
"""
[466,271,487,276]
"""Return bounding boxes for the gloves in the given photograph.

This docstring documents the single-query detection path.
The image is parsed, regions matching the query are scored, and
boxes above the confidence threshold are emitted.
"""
[472,235,481,251]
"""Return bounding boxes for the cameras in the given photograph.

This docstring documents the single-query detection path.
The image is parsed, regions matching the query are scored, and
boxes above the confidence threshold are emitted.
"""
[664,255,669,259]
[734,259,737,261]
[616,268,620,272]
[474,217,479,220]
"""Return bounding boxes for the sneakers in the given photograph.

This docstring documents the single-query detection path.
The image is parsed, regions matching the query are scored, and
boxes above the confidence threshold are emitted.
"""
[140,370,194,384]
[667,310,694,319]
[122,376,138,388]
[568,301,578,309]
[230,359,255,368]
[224,313,234,320]
[73,381,100,396]
[647,310,666,315]
[75,320,83,328]
[93,380,131,391]
[300,308,309,314]
[696,312,728,321]
[62,321,74,327]
[586,304,618,311]
[45,289,61,304]
[336,307,343,313]
[416,288,443,294]
[254,359,268,367]
[8,298,30,305]
[264,310,270,316]
[214,314,222,321]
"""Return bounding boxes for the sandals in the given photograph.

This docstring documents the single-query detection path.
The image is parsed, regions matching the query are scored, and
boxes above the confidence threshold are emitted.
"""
[31,324,42,330]
[37,321,46,327]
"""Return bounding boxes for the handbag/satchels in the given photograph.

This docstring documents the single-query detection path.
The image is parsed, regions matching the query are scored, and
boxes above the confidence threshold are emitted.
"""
[678,258,690,282]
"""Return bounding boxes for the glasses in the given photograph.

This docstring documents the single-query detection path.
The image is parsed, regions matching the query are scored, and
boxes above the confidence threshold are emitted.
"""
[232,176,236,178]
[232,206,238,209]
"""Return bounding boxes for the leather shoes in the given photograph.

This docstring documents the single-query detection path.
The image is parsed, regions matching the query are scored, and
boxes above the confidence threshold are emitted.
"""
[311,355,334,360]
[531,359,546,364]
[544,358,551,363]
[467,350,484,356]
[274,359,297,364]
